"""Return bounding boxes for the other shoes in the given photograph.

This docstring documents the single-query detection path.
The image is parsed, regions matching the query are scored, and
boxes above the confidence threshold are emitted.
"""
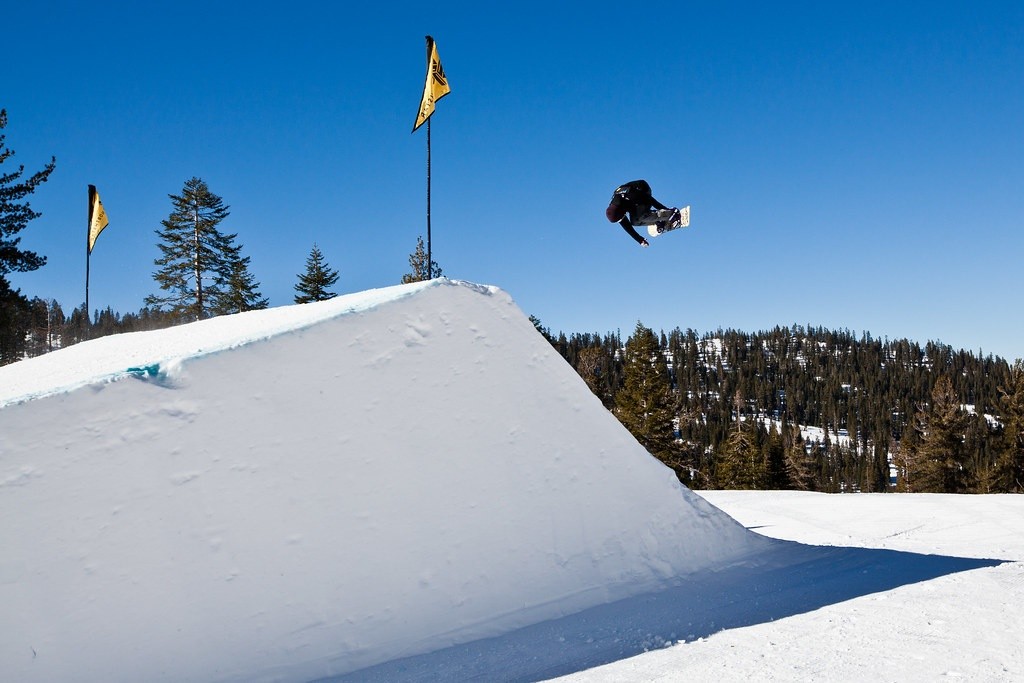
[655,213,665,233]
[669,208,680,228]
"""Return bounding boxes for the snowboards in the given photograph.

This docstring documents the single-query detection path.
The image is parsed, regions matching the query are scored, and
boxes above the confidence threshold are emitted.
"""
[647,206,692,238]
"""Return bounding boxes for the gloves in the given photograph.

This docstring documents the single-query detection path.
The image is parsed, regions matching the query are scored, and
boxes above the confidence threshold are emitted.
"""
[641,239,649,248]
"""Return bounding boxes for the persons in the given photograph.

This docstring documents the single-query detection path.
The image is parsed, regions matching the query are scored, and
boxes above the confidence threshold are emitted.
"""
[603,180,681,247]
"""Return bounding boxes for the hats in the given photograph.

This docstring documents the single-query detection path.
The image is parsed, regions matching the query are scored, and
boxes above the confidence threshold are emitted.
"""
[606,205,624,223]
